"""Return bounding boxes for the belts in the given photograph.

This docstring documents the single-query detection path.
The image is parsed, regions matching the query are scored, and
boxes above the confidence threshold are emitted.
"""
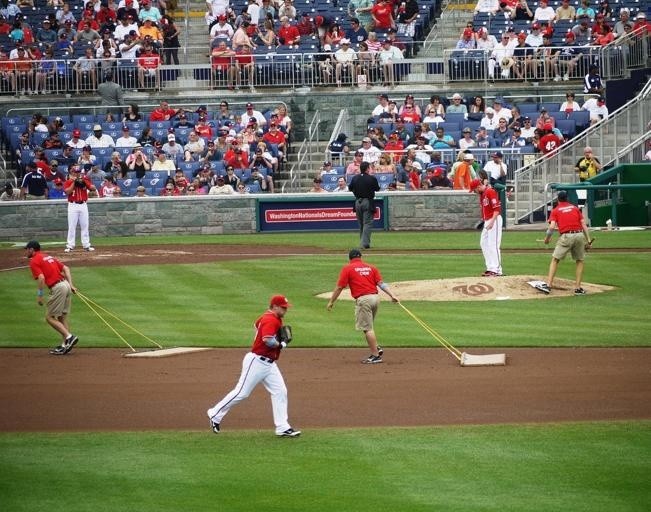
[260,357,273,363]
[70,200,83,204]
[568,230,583,233]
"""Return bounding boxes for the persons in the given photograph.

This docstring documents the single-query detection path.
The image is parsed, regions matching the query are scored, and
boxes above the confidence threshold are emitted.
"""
[534,189,593,297]
[347,162,380,250]
[61,166,96,253]
[21,240,79,356]
[1,0,182,115]
[2,101,294,200]
[451,1,650,87]
[307,64,651,195]
[205,294,301,439]
[204,1,420,91]
[467,178,503,277]
[325,249,398,365]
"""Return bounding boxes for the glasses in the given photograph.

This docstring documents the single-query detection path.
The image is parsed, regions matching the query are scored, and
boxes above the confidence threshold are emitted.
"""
[23,105,275,194]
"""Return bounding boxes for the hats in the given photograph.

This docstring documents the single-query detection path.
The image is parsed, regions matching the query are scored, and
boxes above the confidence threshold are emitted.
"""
[314,94,606,194]
[218,0,397,52]
[271,113,279,126]
[24,240,40,249]
[349,249,361,258]
[271,295,292,307]
[463,12,646,68]
[0,0,152,55]
[246,103,253,108]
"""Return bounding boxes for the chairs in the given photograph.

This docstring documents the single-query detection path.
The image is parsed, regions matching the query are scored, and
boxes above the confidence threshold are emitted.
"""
[321,97,596,191]
[2,1,176,83]
[449,0,651,78]
[2,108,286,197]
[202,0,442,85]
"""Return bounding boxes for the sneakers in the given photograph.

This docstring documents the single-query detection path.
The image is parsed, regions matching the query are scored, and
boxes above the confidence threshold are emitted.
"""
[369,347,383,357]
[282,429,301,436]
[210,420,220,434]
[487,74,569,83]
[49,346,66,355]
[482,271,502,276]
[536,284,551,292]
[574,288,586,294]
[64,247,95,253]
[361,355,383,364]
[65,335,78,352]
[0,86,159,96]
